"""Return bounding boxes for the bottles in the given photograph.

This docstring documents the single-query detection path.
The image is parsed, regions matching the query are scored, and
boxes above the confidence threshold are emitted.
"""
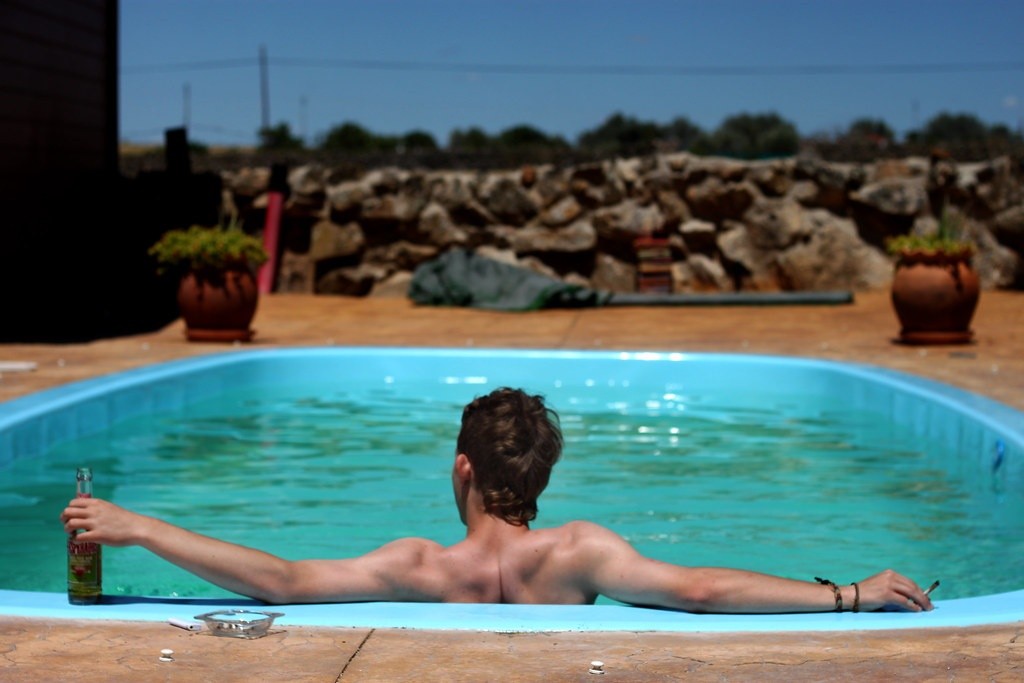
[66,466,102,605]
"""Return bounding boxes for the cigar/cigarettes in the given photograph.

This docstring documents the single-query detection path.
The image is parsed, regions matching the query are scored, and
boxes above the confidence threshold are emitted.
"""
[914,579,940,604]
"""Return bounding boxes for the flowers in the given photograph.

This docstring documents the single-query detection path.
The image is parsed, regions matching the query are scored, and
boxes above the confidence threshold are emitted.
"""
[145,225,273,276]
[883,226,983,255]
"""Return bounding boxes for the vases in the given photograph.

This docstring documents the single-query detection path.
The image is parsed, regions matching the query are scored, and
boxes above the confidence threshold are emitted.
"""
[888,251,984,348]
[179,271,260,345]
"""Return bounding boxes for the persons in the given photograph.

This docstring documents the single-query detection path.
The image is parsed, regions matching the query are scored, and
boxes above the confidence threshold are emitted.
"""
[60,386,935,613]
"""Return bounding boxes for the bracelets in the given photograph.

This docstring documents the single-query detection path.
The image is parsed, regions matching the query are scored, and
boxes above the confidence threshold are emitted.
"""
[850,581,860,613]
[814,575,843,611]
